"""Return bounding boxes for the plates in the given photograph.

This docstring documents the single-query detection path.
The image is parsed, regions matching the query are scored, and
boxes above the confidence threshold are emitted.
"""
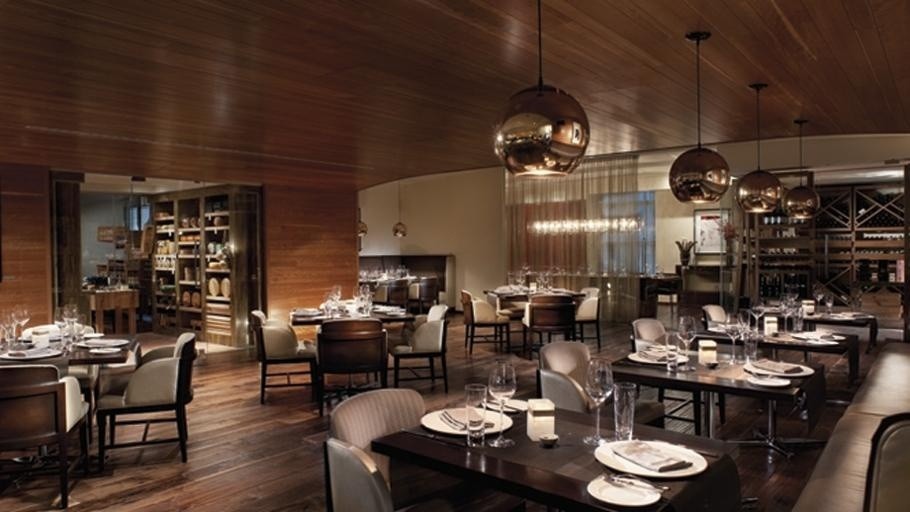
[0,333,130,360]
[587,478,663,507]
[291,312,322,317]
[423,407,514,436]
[747,374,792,386]
[742,361,815,377]
[628,350,691,365]
[480,399,528,413]
[594,439,709,477]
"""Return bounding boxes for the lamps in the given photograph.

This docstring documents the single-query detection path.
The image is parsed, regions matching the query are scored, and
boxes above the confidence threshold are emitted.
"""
[358,208,368,236]
[670,33,730,204]
[492,1,590,175]
[781,118,820,220]
[392,180,406,238]
[737,83,783,213]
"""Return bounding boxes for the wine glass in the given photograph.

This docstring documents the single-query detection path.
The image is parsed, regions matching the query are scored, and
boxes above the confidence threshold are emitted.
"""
[696,292,846,368]
[677,315,697,373]
[0,304,79,352]
[507,271,549,296]
[583,358,615,446]
[487,360,518,447]
[324,285,373,320]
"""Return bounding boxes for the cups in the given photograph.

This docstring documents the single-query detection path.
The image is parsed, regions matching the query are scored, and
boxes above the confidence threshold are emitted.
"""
[612,381,637,440]
[464,384,486,449]
[665,332,684,371]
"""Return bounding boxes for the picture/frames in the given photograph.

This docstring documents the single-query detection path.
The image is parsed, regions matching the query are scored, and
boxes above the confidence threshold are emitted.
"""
[693,208,730,254]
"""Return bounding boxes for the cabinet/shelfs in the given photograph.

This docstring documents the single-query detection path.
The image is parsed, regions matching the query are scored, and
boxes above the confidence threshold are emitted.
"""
[148,186,265,350]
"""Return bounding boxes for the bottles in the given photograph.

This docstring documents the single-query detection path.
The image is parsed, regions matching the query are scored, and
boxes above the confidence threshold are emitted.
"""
[760,248,801,271]
[764,216,807,239]
[760,274,806,298]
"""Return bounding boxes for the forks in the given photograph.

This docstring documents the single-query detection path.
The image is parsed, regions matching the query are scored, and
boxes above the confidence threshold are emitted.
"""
[440,410,495,431]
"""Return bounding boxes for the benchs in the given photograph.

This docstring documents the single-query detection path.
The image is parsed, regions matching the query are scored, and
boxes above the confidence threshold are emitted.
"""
[359,254,455,308]
